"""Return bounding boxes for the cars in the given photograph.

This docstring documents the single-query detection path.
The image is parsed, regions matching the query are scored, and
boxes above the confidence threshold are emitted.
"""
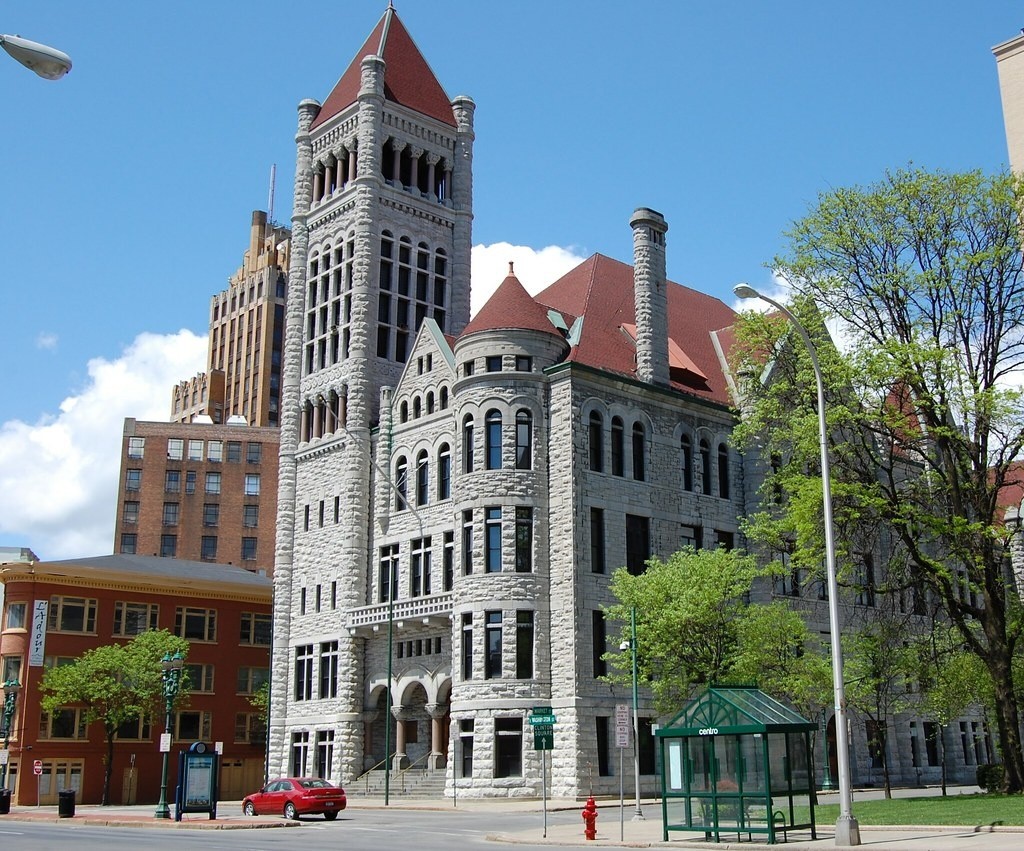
[242,777,348,821]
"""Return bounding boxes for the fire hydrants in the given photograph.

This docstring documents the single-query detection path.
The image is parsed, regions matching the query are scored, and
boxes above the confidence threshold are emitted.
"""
[581,797,599,841]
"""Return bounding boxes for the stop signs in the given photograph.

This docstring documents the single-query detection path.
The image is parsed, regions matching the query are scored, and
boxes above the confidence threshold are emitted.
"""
[34,760,43,776]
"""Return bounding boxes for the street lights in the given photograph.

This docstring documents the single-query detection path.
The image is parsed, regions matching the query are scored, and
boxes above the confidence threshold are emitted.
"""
[733,282,862,846]
[0,676,22,788]
[620,639,646,821]
[153,647,184,820]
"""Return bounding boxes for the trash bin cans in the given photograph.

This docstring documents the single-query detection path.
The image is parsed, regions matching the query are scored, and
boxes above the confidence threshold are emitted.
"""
[58,789,75,819]
[0,788,11,814]
